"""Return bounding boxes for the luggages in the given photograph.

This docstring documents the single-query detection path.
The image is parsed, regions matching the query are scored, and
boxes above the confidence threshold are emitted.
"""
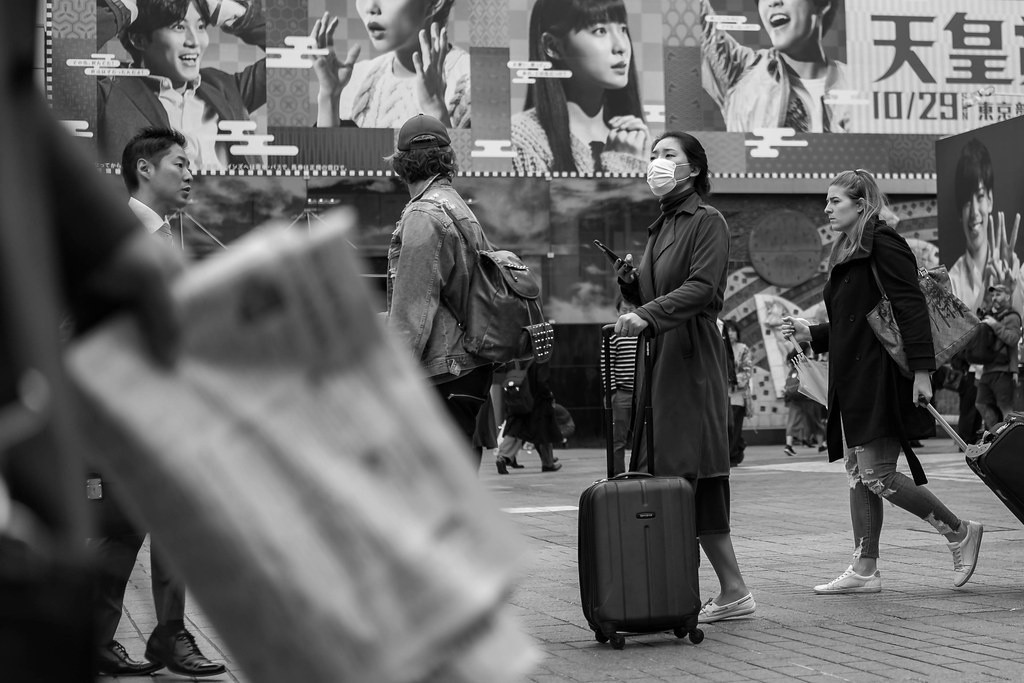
[579,323,704,650]
[917,395,1023,523]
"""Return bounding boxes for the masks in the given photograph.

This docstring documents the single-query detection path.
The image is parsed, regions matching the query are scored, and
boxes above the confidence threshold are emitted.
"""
[647,159,690,196]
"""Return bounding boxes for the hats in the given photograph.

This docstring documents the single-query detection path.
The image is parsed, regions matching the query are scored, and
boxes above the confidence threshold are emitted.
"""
[398,114,451,151]
[989,284,1011,295]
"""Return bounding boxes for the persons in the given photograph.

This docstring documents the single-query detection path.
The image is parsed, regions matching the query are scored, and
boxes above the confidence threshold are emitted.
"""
[725,320,750,465]
[378,113,495,469]
[0,0,174,683]
[510,0,656,175]
[97,0,265,166]
[98,126,228,675]
[957,346,977,451]
[784,342,828,455]
[782,170,984,594]
[310,0,469,129]
[615,130,754,624]
[699,0,852,132]
[950,141,1023,380]
[601,296,638,473]
[975,285,1021,425]
[495,364,575,474]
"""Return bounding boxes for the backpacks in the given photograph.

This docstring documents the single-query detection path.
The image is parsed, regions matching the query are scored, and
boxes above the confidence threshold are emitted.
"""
[414,198,554,363]
[783,369,808,403]
[500,361,533,419]
[965,312,1022,365]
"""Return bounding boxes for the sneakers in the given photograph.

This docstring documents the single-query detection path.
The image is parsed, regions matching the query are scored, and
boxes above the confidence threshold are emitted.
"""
[698,593,756,623]
[946,521,983,587]
[814,565,881,594]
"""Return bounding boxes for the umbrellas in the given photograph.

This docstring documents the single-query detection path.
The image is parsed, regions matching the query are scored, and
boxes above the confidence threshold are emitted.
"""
[790,319,828,408]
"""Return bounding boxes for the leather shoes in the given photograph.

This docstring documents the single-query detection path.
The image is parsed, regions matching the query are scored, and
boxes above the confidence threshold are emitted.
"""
[99,640,165,676]
[144,626,225,676]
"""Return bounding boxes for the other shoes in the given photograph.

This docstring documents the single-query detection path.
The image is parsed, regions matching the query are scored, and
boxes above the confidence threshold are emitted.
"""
[542,464,562,472]
[553,457,558,462]
[496,460,509,474]
[784,445,795,456]
[507,458,524,468]
[802,441,814,448]
[819,445,827,451]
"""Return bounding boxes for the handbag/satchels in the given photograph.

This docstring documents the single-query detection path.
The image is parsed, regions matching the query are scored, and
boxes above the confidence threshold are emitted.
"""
[743,384,753,417]
[865,265,982,377]
[551,402,575,437]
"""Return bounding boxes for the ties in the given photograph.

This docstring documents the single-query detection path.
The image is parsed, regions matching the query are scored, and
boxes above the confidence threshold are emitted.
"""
[158,223,172,244]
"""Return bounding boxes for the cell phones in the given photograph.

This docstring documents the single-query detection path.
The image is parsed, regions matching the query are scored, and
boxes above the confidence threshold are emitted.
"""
[593,239,634,274]
